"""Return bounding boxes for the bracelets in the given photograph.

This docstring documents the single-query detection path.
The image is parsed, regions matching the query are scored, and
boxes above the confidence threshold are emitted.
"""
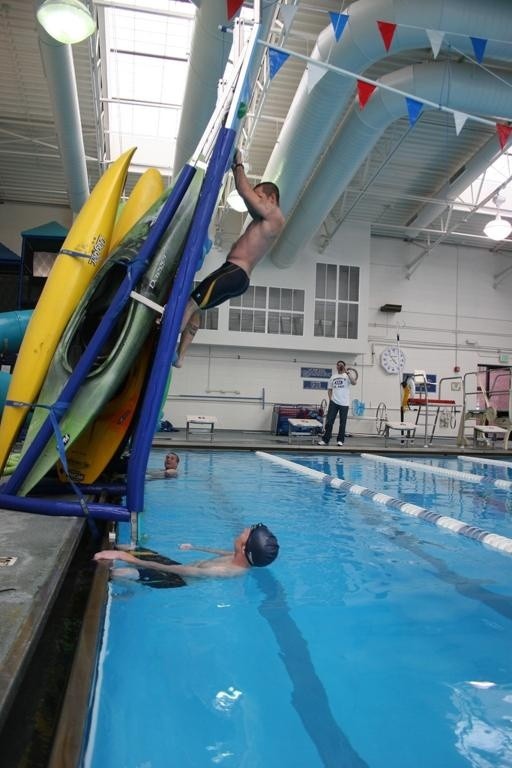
[235,164,244,170]
[347,372,350,375]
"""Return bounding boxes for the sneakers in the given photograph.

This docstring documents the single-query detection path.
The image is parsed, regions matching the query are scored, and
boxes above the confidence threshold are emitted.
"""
[317,439,344,446]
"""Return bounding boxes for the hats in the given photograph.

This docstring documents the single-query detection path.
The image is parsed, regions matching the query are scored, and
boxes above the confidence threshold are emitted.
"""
[246,526,279,567]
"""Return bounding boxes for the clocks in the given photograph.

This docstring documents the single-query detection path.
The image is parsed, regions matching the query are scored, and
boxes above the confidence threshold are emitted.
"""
[381,347,407,374]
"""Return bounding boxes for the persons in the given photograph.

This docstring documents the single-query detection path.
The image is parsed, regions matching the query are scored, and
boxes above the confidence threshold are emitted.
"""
[171,146,286,369]
[93,523,280,601]
[145,452,180,479]
[317,360,357,446]
